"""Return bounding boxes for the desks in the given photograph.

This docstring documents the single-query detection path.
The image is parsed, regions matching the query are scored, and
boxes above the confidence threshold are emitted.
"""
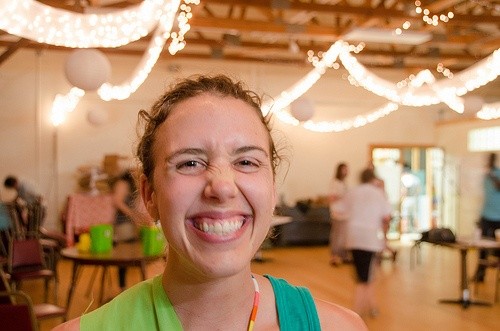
[60,244,156,322]
[424,236,500,309]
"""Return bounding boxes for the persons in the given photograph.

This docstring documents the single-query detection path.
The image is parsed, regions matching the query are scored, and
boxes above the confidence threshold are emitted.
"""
[4,177,47,266]
[328,157,399,317]
[472,151,500,281]
[110,166,144,292]
[52,73,367,331]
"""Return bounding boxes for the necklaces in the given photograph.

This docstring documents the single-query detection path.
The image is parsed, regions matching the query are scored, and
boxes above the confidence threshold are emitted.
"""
[247,274,262,330]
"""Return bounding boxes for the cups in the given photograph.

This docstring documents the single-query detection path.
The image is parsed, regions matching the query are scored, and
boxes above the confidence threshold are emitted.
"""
[90,224,114,253]
[141,225,164,255]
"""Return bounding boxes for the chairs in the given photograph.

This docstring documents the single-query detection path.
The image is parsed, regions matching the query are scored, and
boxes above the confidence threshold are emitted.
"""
[0,195,71,331]
